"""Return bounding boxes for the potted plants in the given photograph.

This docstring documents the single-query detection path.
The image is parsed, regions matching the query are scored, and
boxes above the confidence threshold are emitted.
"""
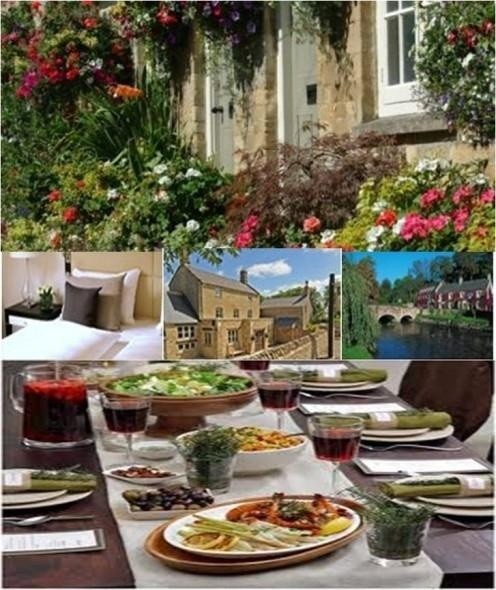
[38,287,54,313]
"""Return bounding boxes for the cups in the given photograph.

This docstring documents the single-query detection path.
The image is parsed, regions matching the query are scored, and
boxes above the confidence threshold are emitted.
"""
[184,454,234,495]
[8,364,93,448]
[363,514,430,568]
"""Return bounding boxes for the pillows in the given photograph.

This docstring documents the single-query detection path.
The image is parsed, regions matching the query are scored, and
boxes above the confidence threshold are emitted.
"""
[69,275,122,331]
[62,282,100,326]
[72,269,139,325]
[0,320,120,362]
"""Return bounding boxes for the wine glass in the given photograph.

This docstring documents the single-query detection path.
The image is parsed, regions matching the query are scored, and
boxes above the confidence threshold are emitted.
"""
[256,369,303,430]
[307,414,364,493]
[99,390,153,467]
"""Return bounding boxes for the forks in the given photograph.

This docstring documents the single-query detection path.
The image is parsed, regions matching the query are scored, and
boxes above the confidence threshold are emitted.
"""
[439,516,494,531]
[357,443,463,453]
[298,390,390,400]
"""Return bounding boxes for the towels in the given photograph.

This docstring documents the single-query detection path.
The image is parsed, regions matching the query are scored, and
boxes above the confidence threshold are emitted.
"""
[88,368,121,381]
[314,410,452,429]
[2,472,96,492]
[261,369,387,383]
[380,478,494,498]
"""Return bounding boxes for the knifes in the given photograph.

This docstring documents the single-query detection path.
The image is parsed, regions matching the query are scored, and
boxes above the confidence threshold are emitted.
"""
[3,512,97,523]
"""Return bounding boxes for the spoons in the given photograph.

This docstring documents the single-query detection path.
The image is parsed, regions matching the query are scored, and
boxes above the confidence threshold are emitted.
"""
[3,514,54,527]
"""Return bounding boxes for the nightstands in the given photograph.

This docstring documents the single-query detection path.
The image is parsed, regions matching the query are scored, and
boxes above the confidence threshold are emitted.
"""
[6,299,62,333]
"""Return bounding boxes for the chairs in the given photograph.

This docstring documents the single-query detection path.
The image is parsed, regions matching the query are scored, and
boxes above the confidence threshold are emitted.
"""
[393,361,493,443]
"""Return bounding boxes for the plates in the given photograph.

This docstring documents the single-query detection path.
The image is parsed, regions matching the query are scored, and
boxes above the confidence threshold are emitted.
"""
[102,464,185,484]
[3,469,95,509]
[300,378,389,392]
[361,422,454,441]
[130,441,177,459]
[120,487,216,520]
[379,475,494,516]
[176,427,308,473]
[144,495,368,573]
